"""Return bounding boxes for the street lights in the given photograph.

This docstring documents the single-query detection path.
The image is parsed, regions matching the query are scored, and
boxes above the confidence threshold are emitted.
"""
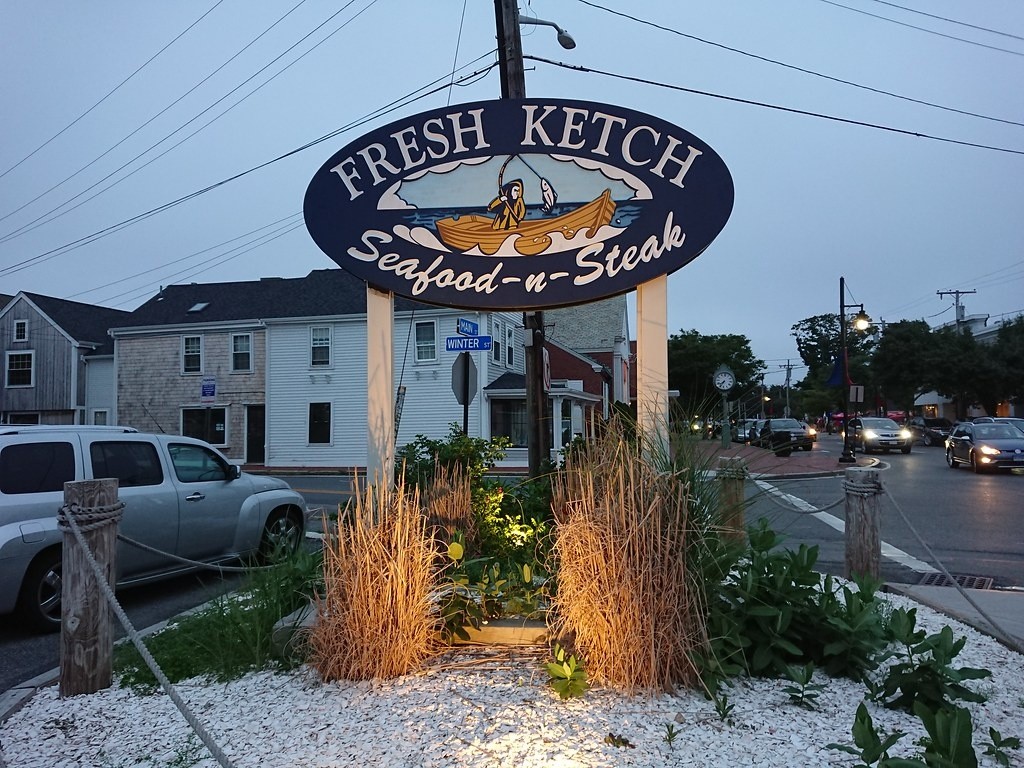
[839,304,869,464]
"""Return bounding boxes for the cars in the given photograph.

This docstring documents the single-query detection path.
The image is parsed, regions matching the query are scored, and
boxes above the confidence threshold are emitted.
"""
[949,417,982,436]
[798,421,816,442]
[749,419,767,447]
[755,418,813,451]
[732,419,759,441]
[972,417,1024,432]
[906,416,953,446]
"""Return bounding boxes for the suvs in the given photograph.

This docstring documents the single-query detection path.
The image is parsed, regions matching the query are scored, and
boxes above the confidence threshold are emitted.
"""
[0,424,309,632]
[945,422,1024,473]
[843,417,912,453]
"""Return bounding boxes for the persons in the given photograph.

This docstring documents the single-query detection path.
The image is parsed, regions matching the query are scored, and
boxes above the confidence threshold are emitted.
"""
[817,417,825,433]
[802,417,806,429]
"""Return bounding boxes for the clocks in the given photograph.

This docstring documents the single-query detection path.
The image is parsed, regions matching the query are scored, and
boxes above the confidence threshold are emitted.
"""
[715,372,734,391]
